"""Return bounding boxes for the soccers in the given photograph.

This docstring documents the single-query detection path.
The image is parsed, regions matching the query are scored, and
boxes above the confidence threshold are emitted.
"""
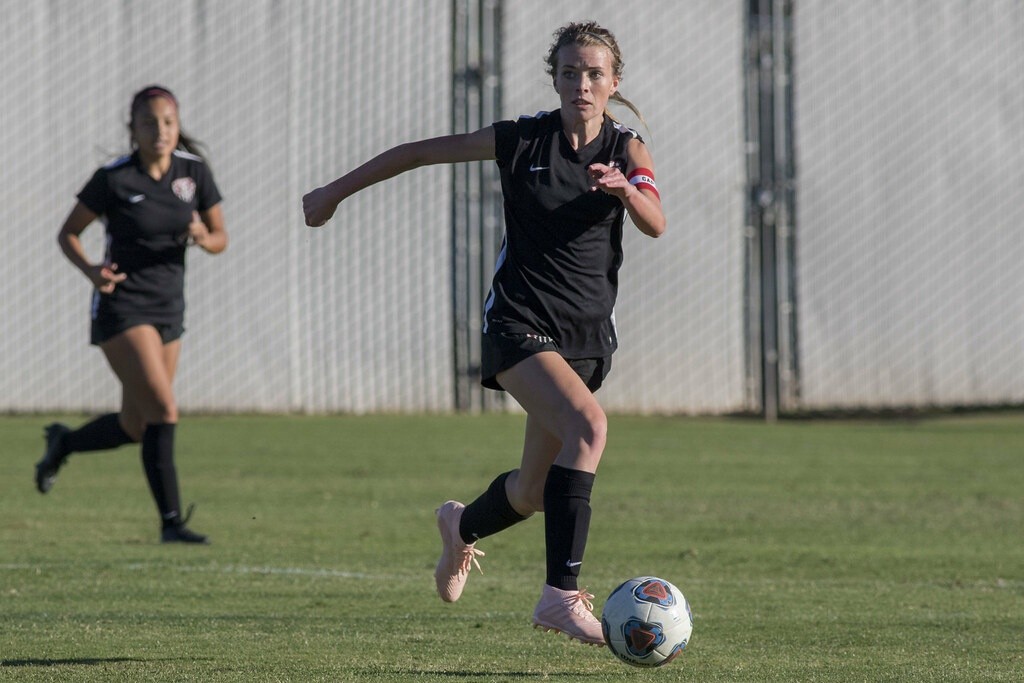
[601,575,696,669]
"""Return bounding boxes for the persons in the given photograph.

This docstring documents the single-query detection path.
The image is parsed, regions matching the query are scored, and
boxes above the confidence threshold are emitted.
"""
[302,22,666,645]
[34,87,228,545]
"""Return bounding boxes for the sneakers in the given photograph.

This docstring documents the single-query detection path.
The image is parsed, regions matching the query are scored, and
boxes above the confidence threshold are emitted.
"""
[35,422,72,495]
[530,582,608,650]
[436,497,486,603]
[159,506,206,542]
